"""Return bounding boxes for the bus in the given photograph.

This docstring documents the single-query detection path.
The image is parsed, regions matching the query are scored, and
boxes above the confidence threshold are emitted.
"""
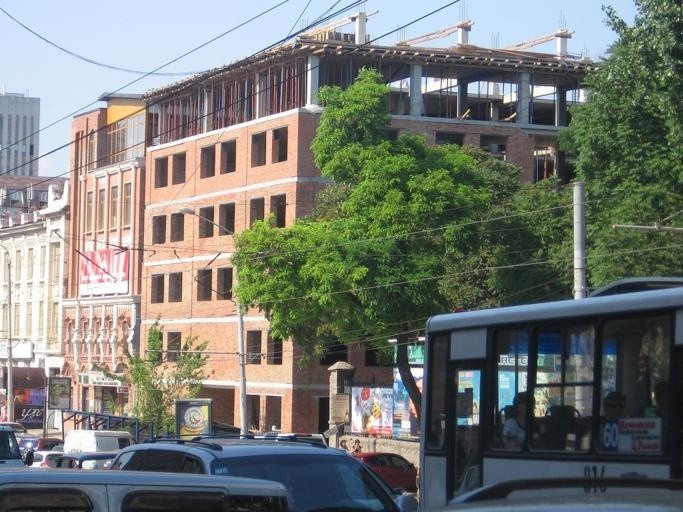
[390,275,683,511]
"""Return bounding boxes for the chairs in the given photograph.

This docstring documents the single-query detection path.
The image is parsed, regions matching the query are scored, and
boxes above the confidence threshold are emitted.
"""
[545,405,582,449]
[496,406,535,451]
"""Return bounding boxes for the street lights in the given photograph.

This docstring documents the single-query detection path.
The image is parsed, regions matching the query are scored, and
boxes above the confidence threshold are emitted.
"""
[181,209,249,440]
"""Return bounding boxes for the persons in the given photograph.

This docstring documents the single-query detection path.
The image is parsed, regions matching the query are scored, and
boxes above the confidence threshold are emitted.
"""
[501,393,537,453]
[599,390,627,454]
[339,439,361,453]
[653,380,672,457]
[0,398,7,422]
